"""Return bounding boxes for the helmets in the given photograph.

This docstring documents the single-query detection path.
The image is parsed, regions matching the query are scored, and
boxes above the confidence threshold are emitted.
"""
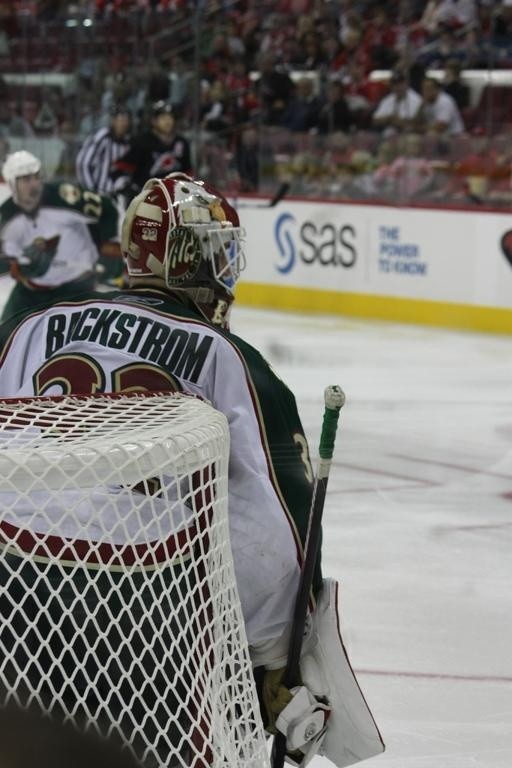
[118,172,240,331]
[106,99,178,137]
[2,150,45,204]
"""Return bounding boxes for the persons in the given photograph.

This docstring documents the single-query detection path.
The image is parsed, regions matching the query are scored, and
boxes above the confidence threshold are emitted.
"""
[74,100,191,256]
[0,0,512,204]
[0,170,334,768]
[0,150,127,351]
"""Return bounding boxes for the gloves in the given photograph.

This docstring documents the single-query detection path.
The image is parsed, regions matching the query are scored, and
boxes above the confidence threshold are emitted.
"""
[92,241,124,284]
[15,237,56,279]
